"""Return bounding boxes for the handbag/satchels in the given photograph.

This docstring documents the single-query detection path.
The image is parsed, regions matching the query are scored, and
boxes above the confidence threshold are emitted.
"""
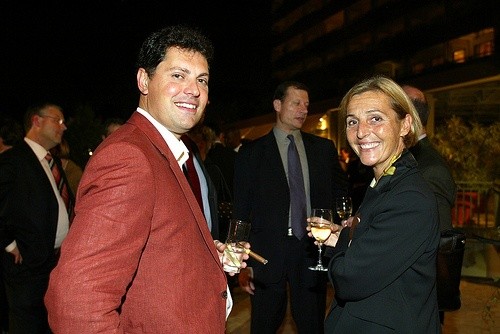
[436,231,466,312]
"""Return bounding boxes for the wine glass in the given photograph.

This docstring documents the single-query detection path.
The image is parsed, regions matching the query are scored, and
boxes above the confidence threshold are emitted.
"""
[337,197,354,228]
[307,207,332,272]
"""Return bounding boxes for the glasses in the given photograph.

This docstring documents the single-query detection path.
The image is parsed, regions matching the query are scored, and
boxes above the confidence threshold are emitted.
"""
[40,115,65,125]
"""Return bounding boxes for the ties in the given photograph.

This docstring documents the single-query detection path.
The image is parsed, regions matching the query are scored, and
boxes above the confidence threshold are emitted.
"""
[45,153,75,229]
[288,134,308,241]
[180,135,204,218]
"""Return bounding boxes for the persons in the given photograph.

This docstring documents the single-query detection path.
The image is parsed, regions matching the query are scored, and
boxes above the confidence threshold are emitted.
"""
[340,83,458,327]
[233,76,348,334]
[42,25,252,333]
[100,116,127,141]
[307,76,444,334]
[0,101,77,333]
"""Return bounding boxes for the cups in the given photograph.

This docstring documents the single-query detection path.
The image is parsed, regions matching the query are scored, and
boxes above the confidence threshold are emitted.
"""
[221,217,251,272]
